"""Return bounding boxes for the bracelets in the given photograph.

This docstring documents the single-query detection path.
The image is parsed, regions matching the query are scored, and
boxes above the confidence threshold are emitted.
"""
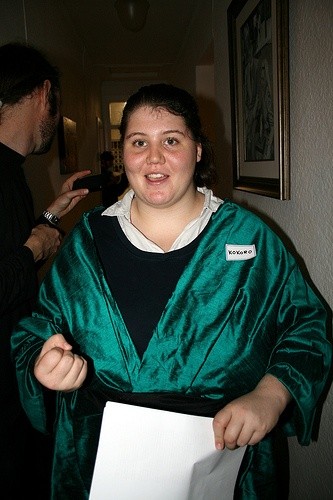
[43,210,61,225]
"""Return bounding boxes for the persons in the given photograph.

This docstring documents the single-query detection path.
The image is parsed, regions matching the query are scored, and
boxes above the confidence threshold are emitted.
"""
[10,82,333,500]
[0,42,89,500]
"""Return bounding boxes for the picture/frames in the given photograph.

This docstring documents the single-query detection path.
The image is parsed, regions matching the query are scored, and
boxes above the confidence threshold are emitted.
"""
[226,0,291,201]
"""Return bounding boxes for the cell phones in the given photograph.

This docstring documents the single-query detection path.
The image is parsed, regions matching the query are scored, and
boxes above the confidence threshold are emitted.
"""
[72,172,111,193]
